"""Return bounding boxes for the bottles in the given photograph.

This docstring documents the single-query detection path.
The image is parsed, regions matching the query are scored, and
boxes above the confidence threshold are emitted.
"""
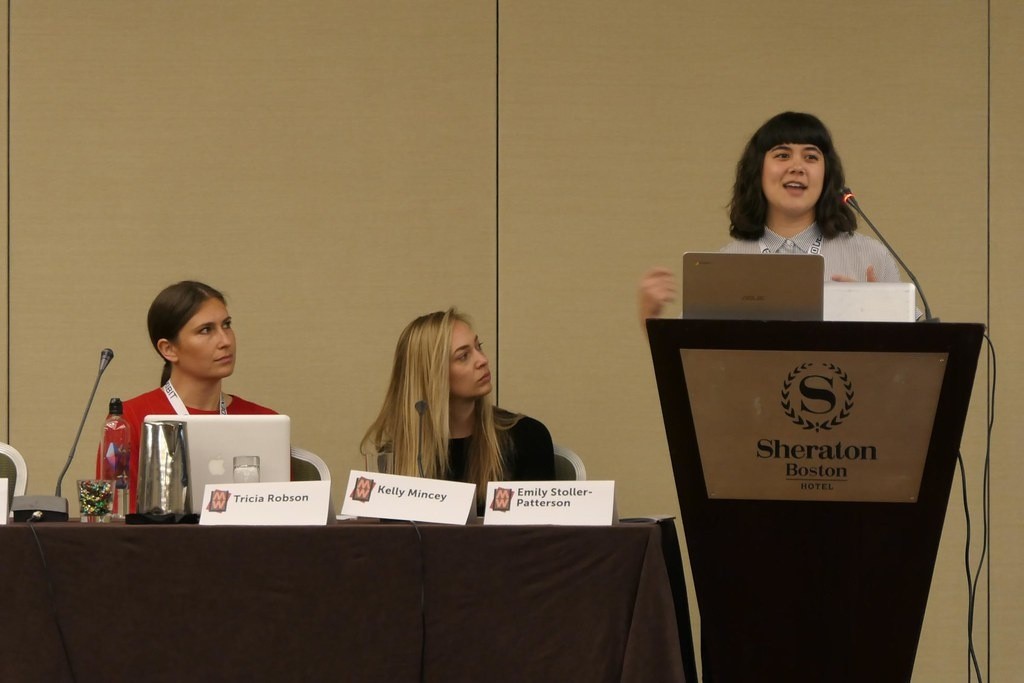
[99,397,130,520]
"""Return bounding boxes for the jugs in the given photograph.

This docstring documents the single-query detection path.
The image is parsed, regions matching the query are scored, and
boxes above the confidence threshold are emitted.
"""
[135,420,194,515]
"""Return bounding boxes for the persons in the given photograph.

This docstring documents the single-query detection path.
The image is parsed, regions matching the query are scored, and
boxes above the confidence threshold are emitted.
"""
[361,310,556,517]
[96,281,293,515]
[640,110,900,328]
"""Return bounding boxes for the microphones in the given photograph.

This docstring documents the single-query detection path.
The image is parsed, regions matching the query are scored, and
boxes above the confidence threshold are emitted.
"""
[415,399,426,479]
[838,187,940,324]
[10,347,115,523]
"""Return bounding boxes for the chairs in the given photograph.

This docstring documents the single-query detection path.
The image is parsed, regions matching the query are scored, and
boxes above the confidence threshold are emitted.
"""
[292,447,332,481]
[0,441,29,514]
[554,442,588,480]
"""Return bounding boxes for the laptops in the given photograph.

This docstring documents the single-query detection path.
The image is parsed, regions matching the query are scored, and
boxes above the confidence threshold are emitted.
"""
[682,252,824,321]
[146,413,291,515]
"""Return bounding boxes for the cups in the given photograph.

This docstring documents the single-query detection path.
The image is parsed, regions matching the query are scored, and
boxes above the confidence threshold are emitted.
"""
[364,451,394,475]
[76,478,116,523]
[233,456,260,484]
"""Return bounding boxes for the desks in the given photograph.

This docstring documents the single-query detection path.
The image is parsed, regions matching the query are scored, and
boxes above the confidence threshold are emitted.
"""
[0,514,699,683]
